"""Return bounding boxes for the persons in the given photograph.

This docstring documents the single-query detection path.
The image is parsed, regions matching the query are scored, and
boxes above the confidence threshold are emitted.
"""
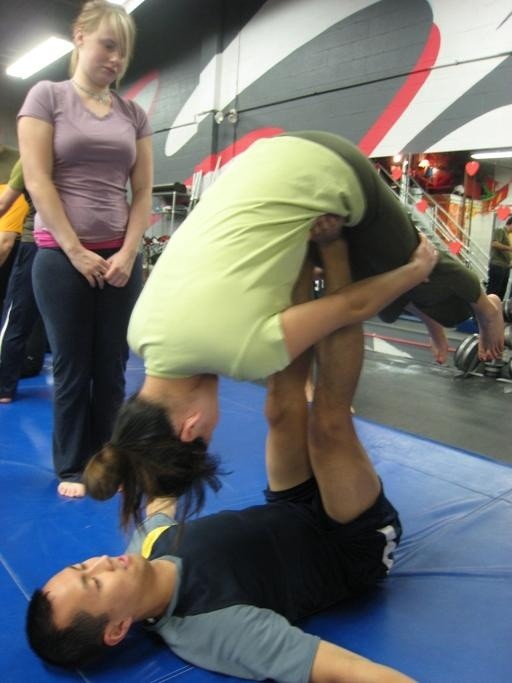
[15,0,154,498]
[0,157,38,403]
[0,184,30,268]
[485,217,512,301]
[26,214,420,683]
[82,129,506,544]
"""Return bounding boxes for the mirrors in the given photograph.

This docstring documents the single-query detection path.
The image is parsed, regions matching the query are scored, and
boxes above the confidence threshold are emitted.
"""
[364,147,511,324]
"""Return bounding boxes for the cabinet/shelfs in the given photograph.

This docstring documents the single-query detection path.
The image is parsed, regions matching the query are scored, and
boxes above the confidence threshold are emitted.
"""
[139,191,195,286]
